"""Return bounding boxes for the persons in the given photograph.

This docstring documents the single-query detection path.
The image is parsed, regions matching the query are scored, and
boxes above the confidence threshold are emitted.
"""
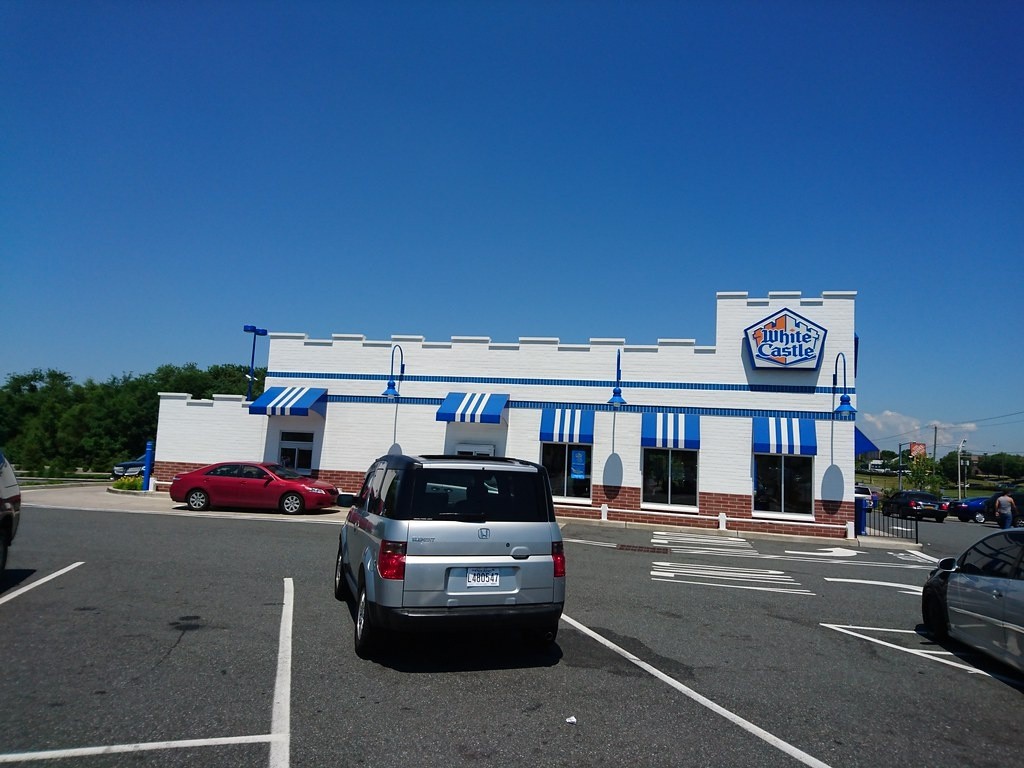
[995,488,1016,530]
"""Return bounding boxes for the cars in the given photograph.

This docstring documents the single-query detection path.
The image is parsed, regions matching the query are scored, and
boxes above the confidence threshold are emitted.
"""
[948,496,997,524]
[169,461,339,515]
[882,491,948,523]
[943,499,951,506]
[112,451,155,480]
[922,527,1024,674]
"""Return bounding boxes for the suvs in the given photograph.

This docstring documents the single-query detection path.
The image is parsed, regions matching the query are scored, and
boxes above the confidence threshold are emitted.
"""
[0,454,22,573]
[989,491,1024,527]
[855,484,874,514]
[334,452,566,658]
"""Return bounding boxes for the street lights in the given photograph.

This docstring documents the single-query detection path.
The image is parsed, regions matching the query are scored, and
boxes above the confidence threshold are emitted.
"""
[242,323,269,402]
[898,441,917,493]
[957,438,968,501]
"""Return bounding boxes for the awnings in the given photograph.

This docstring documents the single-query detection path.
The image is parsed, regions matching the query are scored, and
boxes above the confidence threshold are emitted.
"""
[436,392,510,426]
[753,416,818,455]
[640,412,701,449]
[539,409,595,444]
[855,425,879,457]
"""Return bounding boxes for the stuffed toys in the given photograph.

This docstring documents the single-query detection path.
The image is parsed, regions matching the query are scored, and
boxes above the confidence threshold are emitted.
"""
[248,387,328,417]
